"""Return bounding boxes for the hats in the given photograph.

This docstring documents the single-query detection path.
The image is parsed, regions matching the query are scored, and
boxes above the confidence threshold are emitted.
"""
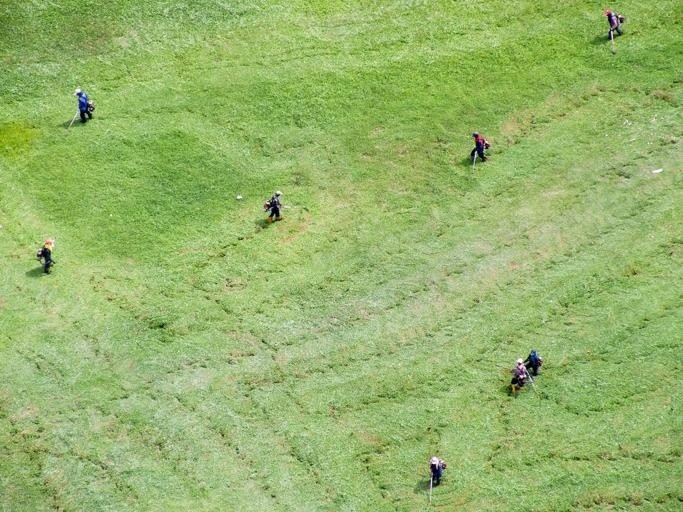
[470,132,479,138]
[45,240,53,244]
[275,190,282,195]
[602,10,611,16]
[428,455,440,465]
[72,88,81,97]
[516,357,524,364]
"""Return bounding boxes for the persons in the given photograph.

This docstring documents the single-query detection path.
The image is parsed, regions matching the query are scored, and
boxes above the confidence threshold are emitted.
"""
[266,191,284,224]
[41,238,54,274]
[509,358,527,393]
[427,456,442,486]
[523,349,540,376]
[603,9,624,42]
[72,88,92,124]
[470,131,488,162]
